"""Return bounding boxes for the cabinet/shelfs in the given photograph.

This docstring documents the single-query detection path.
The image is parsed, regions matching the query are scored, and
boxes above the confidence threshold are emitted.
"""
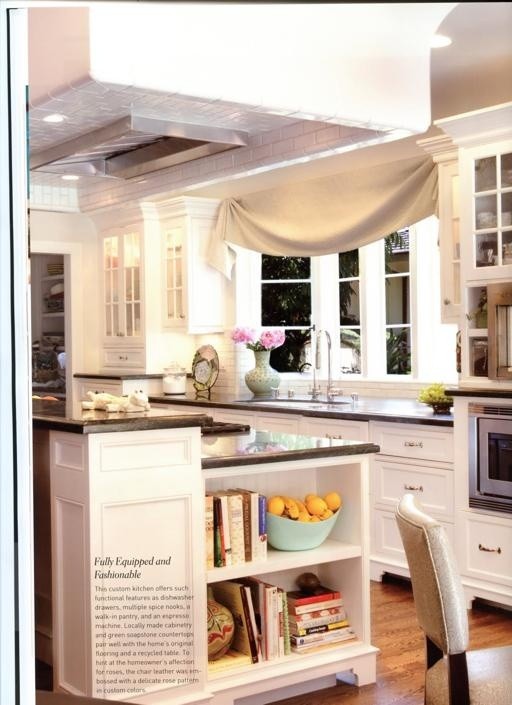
[31,255,64,354]
[74,379,150,418]
[78,215,226,374]
[437,136,512,392]
[201,454,377,705]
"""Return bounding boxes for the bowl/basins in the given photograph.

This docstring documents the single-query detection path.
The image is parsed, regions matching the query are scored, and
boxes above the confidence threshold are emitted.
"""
[265,503,343,552]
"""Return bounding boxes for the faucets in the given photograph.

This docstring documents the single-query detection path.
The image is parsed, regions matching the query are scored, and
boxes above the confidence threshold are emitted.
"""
[315,328,343,402]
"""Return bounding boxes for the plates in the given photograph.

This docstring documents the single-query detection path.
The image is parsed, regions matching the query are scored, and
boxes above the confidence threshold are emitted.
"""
[192,343,219,391]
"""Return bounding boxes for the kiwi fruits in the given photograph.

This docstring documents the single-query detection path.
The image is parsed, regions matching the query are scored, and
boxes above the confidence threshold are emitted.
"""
[296,573,320,593]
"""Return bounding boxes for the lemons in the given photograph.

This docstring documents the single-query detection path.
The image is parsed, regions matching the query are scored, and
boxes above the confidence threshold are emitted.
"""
[268,491,342,523]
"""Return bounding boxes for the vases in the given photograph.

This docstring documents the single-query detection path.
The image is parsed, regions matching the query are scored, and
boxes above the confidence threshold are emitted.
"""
[244,352,280,401]
[430,403,453,415]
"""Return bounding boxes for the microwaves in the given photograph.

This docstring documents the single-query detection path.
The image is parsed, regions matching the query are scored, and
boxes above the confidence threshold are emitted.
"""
[468,401,512,514]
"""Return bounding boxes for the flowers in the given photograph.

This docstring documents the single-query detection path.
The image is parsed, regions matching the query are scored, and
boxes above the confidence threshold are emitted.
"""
[229,327,285,352]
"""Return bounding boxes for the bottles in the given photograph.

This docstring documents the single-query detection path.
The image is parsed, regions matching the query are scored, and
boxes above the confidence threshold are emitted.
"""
[162,367,187,395]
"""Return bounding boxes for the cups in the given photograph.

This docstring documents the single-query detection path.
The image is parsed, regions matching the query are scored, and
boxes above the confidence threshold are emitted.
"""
[477,211,496,230]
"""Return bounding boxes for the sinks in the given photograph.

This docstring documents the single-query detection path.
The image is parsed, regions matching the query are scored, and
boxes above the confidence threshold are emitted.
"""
[235,398,349,405]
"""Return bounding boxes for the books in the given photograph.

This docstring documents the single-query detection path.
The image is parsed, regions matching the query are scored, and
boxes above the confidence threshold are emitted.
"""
[204,487,359,676]
